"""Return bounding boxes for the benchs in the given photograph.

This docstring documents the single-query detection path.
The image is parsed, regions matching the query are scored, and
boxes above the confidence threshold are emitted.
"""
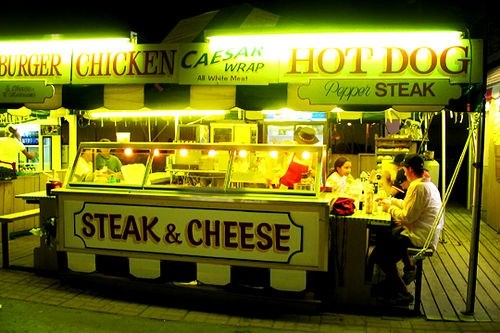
[407,248,433,317]
[0,208,40,269]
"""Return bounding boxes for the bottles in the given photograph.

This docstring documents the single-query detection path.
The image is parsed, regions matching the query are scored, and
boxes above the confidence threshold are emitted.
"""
[359,193,363,210]
[21,131,39,145]
[365,188,373,213]
[373,176,378,193]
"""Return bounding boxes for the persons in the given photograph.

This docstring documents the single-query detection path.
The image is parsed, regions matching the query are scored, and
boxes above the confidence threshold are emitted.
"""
[375,153,445,304]
[386,153,417,284]
[73,148,96,182]
[96,139,126,183]
[266,127,320,189]
[0,132,35,180]
[325,157,353,194]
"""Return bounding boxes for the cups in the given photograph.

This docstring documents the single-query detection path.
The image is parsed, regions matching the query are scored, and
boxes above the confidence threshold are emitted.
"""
[371,202,378,216]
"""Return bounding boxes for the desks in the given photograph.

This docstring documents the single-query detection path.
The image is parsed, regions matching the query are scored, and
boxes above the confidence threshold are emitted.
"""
[329,193,394,304]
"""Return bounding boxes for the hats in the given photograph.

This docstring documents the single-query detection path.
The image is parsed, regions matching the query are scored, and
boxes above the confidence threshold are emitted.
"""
[401,154,429,171]
[294,125,319,145]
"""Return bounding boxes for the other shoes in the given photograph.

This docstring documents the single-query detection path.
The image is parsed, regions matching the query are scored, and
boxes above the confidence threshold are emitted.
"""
[386,292,413,306]
[401,264,417,284]
